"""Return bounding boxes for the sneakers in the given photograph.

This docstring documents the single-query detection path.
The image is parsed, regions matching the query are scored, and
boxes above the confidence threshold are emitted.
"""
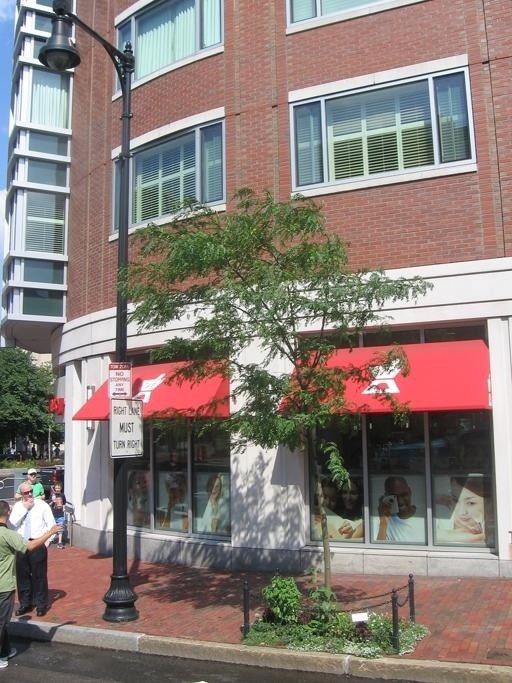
[57,544,64,549]
[0,648,17,668]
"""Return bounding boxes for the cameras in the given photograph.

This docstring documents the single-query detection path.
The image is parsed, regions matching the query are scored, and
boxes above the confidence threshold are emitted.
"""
[385,494,400,515]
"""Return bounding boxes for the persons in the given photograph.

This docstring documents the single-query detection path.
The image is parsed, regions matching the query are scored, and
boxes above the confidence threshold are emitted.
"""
[15,468,45,501]
[127,471,151,528]
[0,500,65,668]
[7,484,57,617]
[32,443,60,459]
[46,480,66,549]
[373,476,426,543]
[156,472,188,530]
[436,477,492,543]
[313,477,365,539]
[197,475,229,533]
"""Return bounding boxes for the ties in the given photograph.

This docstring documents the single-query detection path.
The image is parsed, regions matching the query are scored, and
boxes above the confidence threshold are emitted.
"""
[24,513,31,541]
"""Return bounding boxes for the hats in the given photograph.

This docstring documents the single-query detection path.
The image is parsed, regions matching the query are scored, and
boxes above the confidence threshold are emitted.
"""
[28,469,37,474]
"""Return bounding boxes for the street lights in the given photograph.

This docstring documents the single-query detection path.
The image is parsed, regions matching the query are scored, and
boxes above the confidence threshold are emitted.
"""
[38,0,139,621]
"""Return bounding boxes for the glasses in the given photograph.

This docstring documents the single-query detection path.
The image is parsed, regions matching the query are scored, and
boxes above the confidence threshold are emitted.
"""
[24,489,33,495]
[28,473,36,476]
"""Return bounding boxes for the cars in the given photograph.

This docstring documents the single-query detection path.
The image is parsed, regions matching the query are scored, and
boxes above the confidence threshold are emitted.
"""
[39,466,64,499]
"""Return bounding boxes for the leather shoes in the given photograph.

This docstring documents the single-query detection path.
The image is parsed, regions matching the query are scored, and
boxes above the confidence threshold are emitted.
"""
[15,606,44,616]
